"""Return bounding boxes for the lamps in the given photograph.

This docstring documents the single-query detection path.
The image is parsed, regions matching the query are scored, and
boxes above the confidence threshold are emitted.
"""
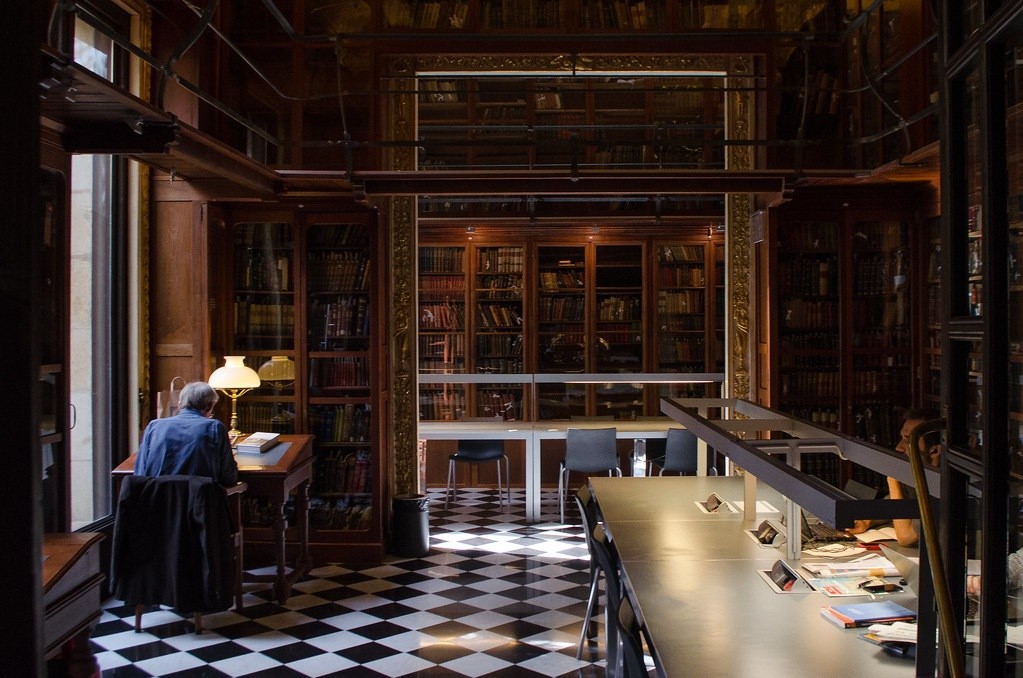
[257,356,294,420]
[209,355,262,437]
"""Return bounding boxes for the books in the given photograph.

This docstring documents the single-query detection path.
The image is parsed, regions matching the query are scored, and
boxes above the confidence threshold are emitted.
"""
[243,498,295,526]
[414,0,731,214]
[318,449,370,530]
[776,222,909,494]
[236,431,281,454]
[240,249,373,291]
[237,403,370,441]
[246,355,369,387]
[794,69,843,115]
[538,243,704,399]
[233,298,371,337]
[923,0,1023,457]
[801,554,1023,647]
[418,247,524,421]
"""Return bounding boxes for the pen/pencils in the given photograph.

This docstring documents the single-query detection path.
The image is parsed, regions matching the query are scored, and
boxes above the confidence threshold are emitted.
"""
[231,447,237,450]
[856,578,875,589]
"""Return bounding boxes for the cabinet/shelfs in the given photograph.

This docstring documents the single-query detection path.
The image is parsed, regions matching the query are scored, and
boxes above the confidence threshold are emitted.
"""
[43,530,107,678]
[222,205,393,564]
[756,160,1023,557]
[410,71,731,173]
[420,218,725,489]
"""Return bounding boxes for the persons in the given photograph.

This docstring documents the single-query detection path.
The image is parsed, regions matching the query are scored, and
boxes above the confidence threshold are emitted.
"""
[967,547,1023,595]
[133,382,240,487]
[846,408,955,547]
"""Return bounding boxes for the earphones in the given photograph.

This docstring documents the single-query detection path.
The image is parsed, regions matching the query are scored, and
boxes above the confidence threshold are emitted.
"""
[929,448,938,455]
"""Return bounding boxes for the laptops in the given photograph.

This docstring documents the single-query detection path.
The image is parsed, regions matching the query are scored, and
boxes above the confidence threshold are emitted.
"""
[801,510,857,543]
[877,544,1023,626]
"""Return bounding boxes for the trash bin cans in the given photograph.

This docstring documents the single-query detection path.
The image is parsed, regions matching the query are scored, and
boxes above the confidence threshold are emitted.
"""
[391,494,431,555]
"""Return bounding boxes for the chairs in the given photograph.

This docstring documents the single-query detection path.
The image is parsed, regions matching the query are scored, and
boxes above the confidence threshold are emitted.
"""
[118,475,247,634]
[575,484,654,678]
[648,428,697,476]
[559,428,624,525]
[446,417,511,511]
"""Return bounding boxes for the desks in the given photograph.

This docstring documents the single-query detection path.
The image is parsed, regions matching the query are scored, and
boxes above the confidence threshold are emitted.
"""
[111,434,318,605]
[588,476,1023,678]
